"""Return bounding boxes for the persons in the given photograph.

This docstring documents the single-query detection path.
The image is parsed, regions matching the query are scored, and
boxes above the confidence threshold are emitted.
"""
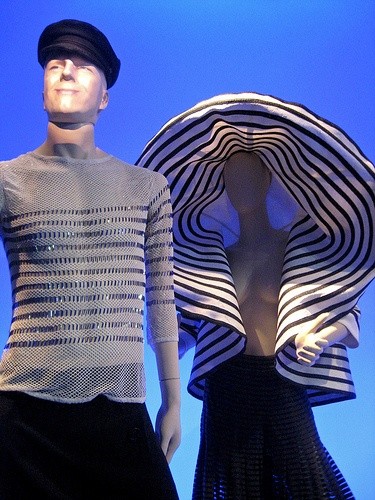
[190,149,358,500]
[0,17,184,500]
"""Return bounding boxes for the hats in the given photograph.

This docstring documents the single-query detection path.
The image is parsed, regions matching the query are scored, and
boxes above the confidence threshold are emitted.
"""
[37,19,122,88]
